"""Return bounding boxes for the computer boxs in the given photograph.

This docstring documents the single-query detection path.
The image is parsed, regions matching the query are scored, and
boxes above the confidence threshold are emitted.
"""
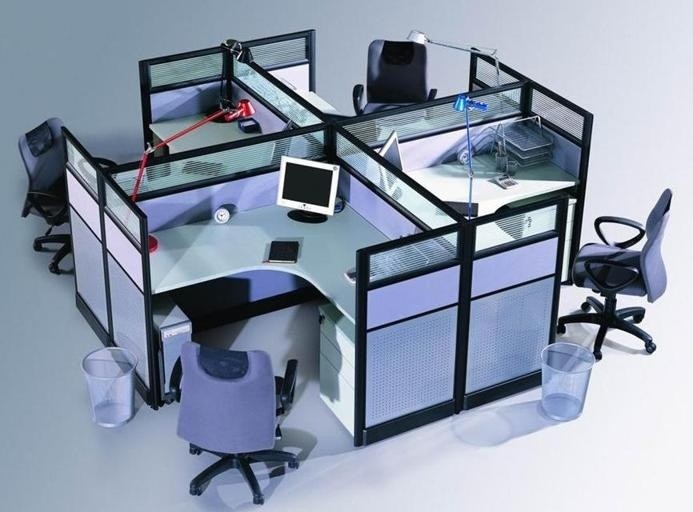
[152,296,193,394]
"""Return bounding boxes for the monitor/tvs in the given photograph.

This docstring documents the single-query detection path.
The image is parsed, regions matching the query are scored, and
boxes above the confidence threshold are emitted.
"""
[276,155,340,224]
[270,120,293,163]
[324,114,377,142]
[378,130,405,201]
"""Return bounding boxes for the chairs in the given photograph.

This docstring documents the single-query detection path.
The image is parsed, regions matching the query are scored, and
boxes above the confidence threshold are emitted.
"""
[556,189,672,360]
[18,117,119,275]
[177,341,298,504]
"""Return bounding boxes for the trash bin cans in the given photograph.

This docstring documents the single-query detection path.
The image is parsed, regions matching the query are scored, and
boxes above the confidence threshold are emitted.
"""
[80,347,138,428]
[540,341,596,422]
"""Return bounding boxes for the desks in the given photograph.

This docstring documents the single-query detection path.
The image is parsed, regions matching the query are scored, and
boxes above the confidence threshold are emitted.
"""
[60,28,593,446]
